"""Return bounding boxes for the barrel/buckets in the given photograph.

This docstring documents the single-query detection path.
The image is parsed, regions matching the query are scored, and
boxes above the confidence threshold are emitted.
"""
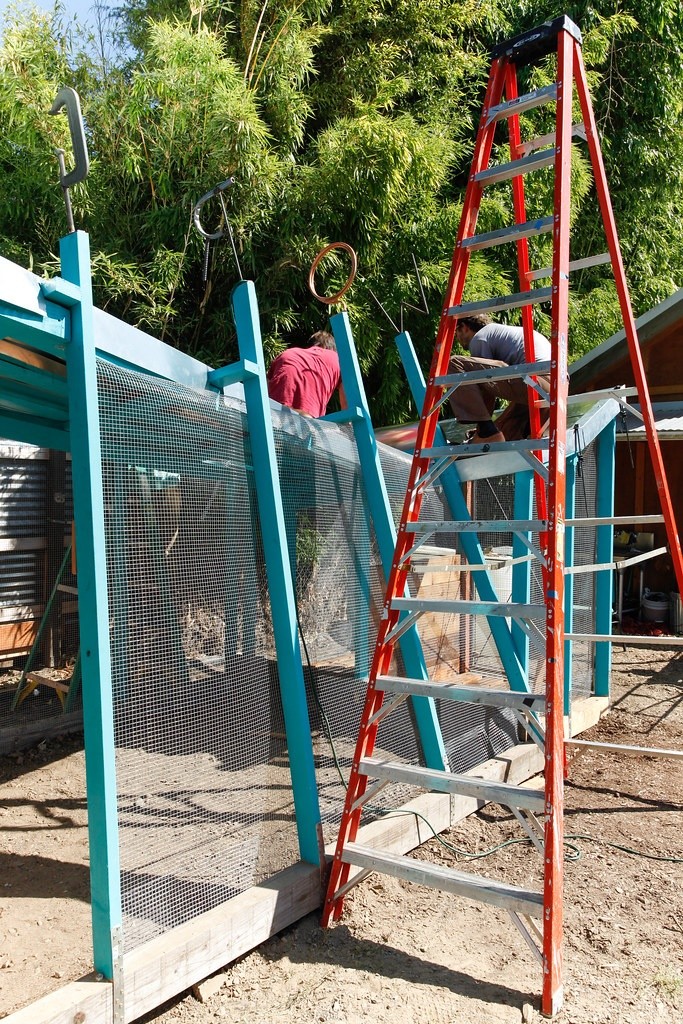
[642,592,671,623]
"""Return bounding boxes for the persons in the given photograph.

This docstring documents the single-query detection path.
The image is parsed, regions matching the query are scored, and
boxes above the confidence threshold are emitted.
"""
[157,476,185,576]
[447,314,570,444]
[268,331,352,426]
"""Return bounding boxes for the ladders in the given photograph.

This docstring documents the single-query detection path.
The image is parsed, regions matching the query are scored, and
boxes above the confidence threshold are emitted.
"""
[320,13,683,1018]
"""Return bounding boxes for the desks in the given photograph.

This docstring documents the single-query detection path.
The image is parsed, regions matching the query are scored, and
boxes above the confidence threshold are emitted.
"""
[613,551,644,625]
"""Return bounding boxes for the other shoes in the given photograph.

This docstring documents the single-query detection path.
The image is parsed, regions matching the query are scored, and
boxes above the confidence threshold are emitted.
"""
[457,431,506,460]
